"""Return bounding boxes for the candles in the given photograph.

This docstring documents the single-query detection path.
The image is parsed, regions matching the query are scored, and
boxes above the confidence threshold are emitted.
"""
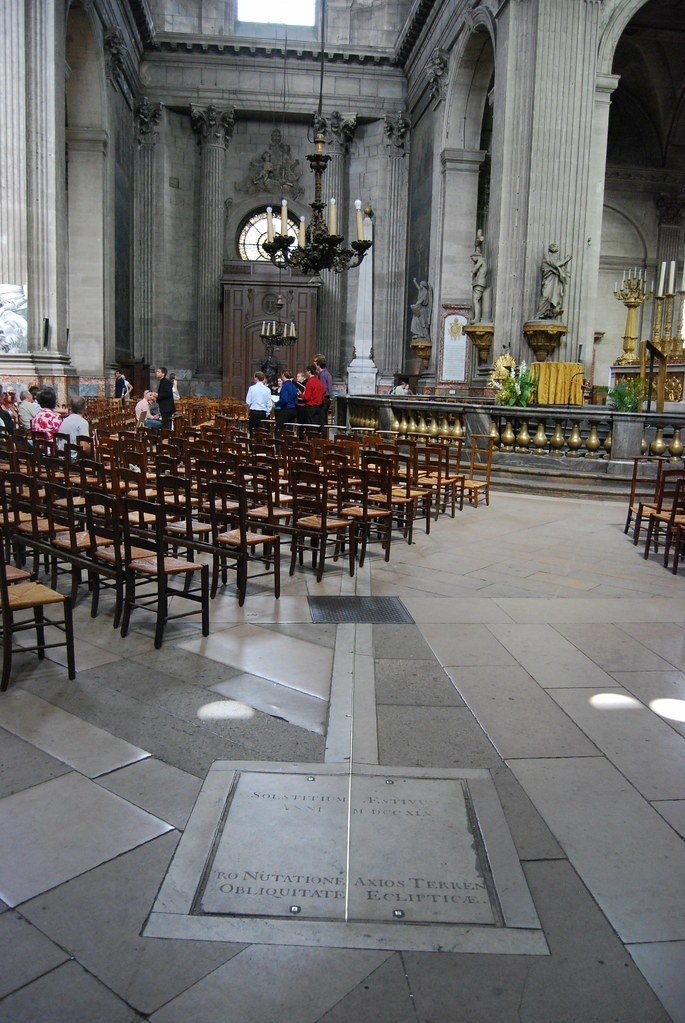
[668,260,676,293]
[614,265,654,296]
[658,261,666,297]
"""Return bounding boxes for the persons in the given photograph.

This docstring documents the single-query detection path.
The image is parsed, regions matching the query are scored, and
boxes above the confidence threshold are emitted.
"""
[115,371,133,405]
[156,367,176,430]
[246,354,333,451]
[411,277,429,339]
[470,252,487,325]
[135,390,162,428]
[396,382,413,395]
[535,243,572,319]
[170,372,180,400]
[0,384,92,462]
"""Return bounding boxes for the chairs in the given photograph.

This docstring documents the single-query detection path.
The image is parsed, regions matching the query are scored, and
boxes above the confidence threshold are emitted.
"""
[0,387,495,695]
[624,456,685,575]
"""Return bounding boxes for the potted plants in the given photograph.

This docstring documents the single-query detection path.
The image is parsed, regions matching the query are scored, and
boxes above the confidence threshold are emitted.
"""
[495,374,537,407]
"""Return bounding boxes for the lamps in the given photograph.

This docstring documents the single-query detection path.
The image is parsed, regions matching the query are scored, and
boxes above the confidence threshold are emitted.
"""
[259,0,374,353]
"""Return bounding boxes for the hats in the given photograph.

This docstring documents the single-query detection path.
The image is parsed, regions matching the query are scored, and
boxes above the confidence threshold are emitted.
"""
[4,386,16,394]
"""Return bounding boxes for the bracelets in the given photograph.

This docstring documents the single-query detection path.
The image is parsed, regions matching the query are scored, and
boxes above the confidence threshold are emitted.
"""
[122,393,124,396]
